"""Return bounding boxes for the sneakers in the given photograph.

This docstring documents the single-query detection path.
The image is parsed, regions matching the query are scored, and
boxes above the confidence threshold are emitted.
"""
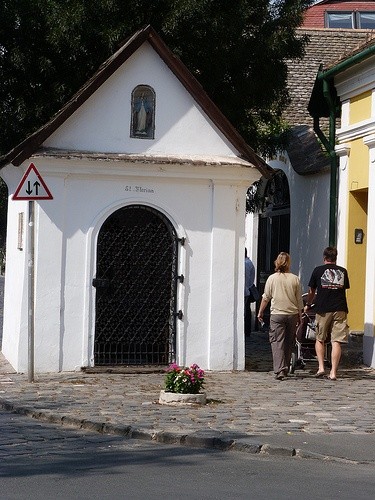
[275,370,288,379]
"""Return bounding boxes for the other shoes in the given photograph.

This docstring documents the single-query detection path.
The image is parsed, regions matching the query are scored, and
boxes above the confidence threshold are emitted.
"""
[246,332,251,337]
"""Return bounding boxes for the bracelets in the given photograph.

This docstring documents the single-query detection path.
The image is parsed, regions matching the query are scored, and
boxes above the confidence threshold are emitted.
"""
[305,302,313,308]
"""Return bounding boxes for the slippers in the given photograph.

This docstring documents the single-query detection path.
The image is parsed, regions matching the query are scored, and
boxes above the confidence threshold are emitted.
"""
[309,372,336,381]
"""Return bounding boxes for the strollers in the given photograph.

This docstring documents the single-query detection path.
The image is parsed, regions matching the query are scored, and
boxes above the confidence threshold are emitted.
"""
[288,291,332,373]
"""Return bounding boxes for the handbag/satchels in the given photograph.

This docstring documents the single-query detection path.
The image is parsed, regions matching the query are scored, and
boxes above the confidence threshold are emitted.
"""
[305,322,318,341]
[248,283,261,302]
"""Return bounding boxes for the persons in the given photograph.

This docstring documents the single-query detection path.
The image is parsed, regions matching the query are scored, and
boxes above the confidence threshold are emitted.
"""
[244,247,255,337]
[304,247,350,381]
[258,252,301,380]
